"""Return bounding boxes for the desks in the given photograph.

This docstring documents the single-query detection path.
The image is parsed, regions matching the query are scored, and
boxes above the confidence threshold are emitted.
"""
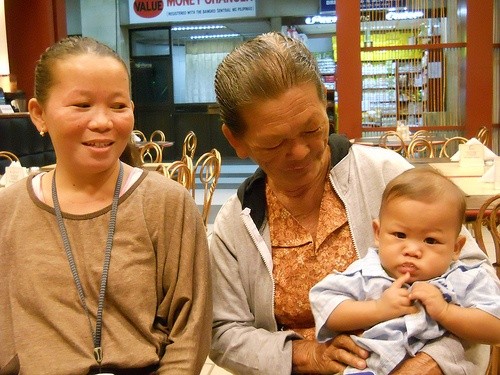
[352,135,444,146]
[0,112,29,118]
[41,163,179,179]
[406,156,500,223]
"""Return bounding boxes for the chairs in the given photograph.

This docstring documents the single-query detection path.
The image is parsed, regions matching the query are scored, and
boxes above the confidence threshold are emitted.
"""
[131,130,222,230]
[381,127,500,278]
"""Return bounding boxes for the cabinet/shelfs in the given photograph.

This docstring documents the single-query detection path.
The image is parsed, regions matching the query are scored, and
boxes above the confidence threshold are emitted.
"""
[321,72,335,124]
[361,72,425,116]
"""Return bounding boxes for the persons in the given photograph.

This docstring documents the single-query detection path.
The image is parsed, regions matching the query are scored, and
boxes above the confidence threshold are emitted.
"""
[209,32,492,375]
[0,35,213,375]
[307,167,500,375]
